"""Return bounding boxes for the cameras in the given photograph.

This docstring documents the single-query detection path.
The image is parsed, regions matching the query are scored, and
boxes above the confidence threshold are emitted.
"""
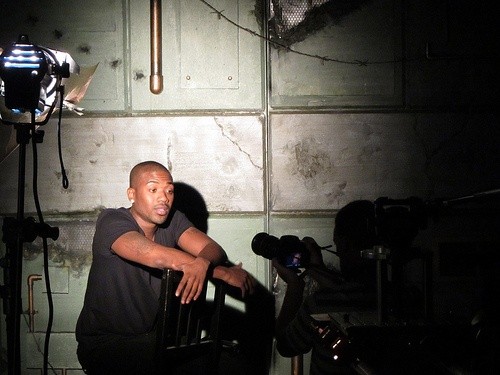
[252,233,312,273]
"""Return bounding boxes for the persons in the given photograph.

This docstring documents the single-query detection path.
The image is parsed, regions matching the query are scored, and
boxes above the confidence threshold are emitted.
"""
[270,197,382,375]
[76,160,257,375]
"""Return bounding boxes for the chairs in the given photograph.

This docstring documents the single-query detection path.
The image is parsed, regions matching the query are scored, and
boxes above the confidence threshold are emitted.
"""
[151,269,233,375]
